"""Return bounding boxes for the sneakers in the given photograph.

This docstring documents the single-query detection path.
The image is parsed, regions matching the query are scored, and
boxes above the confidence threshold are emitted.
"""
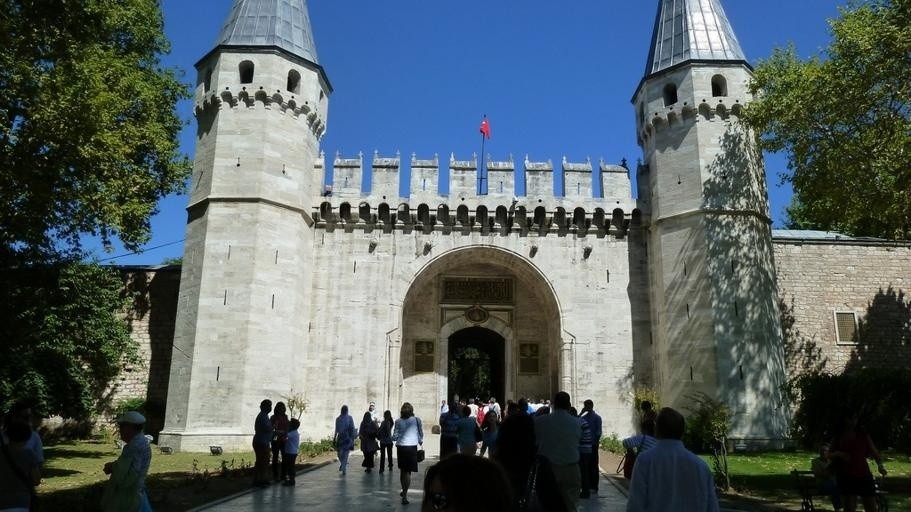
[283,482,290,485]
[403,497,410,504]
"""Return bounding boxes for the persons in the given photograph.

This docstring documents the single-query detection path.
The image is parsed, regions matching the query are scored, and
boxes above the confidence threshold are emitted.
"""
[639,401,657,423]
[103,411,153,512]
[623,420,657,463]
[252,400,278,489]
[0,401,44,463]
[828,409,887,512]
[279,419,300,486]
[359,391,601,512]
[336,406,355,475]
[270,402,290,482]
[812,445,843,512]
[626,407,721,512]
[0,422,43,512]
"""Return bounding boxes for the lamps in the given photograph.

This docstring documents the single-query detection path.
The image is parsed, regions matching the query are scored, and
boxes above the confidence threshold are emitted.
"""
[210,447,222,456]
[160,449,172,453]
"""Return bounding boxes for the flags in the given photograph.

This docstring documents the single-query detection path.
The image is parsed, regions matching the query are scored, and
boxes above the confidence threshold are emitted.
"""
[480,118,490,139]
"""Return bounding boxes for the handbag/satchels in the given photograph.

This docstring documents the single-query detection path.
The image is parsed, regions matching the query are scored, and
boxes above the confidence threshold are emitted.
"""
[477,431,485,441]
[624,448,637,479]
[417,449,425,462]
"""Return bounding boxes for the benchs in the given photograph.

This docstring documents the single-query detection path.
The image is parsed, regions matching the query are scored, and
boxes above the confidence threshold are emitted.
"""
[788,468,908,511]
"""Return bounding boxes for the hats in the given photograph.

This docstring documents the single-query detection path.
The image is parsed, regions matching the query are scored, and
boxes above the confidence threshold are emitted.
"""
[370,401,376,406]
[117,411,147,425]
